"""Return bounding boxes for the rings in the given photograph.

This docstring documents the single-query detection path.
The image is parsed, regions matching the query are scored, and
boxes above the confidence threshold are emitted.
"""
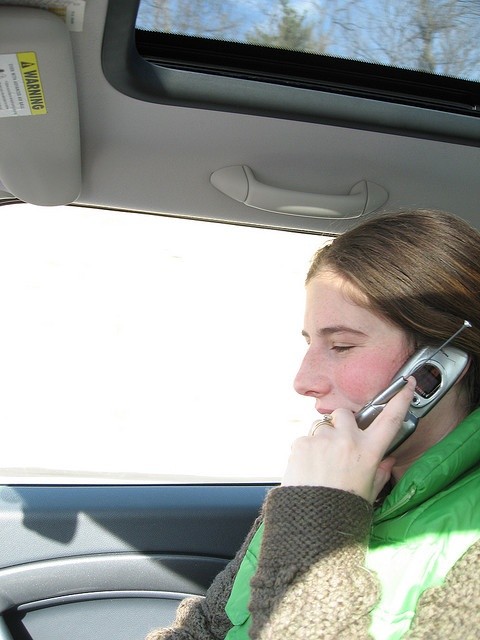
[309,415,335,435]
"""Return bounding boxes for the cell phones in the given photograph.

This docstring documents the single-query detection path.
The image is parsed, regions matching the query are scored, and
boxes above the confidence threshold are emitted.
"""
[350,319,472,462]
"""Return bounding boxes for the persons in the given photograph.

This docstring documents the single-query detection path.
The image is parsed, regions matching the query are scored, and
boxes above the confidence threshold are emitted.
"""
[146,209,480,640]
